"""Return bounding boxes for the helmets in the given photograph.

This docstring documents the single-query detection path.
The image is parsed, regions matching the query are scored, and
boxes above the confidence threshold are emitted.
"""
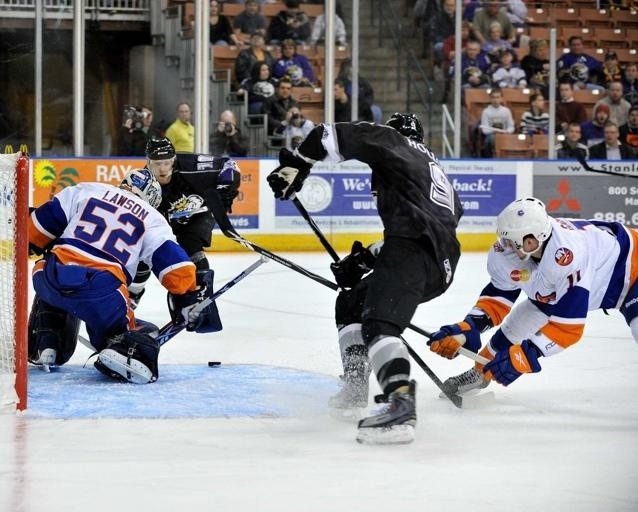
[118,168,163,210]
[386,112,425,143]
[495,197,553,270]
[281,39,295,54]
[284,66,303,86]
[465,67,483,79]
[144,134,176,160]
[569,63,589,83]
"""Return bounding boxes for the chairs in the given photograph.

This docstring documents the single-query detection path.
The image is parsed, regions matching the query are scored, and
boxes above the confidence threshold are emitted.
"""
[525,4,551,25]
[496,128,540,158]
[212,45,243,88]
[529,26,561,52]
[583,8,610,32]
[614,11,637,29]
[556,4,580,28]
[590,33,624,55]
[466,86,500,128]
[501,86,535,128]
[560,32,591,51]
[292,84,325,126]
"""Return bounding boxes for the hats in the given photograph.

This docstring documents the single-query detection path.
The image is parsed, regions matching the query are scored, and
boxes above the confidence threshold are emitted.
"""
[252,28,267,37]
[606,52,617,59]
[596,103,610,115]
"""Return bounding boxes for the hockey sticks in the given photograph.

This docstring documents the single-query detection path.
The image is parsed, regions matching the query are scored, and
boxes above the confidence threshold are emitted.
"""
[78,256,269,355]
[575,146,638,179]
[273,172,495,410]
[202,188,493,365]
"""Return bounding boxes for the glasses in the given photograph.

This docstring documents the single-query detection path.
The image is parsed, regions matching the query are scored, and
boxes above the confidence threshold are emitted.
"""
[291,112,303,118]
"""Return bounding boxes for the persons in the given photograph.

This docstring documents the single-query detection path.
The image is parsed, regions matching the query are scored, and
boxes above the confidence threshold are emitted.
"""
[335,57,373,105]
[480,21,515,70]
[618,108,638,144]
[274,38,313,88]
[266,112,465,446]
[127,135,239,332]
[166,103,194,151]
[491,49,528,88]
[268,0,312,40]
[588,123,638,160]
[235,32,275,85]
[237,61,281,123]
[332,79,374,123]
[233,1,269,45]
[274,107,314,153]
[520,92,551,135]
[624,63,638,102]
[555,76,586,130]
[442,22,476,72]
[209,111,249,156]
[209,1,240,48]
[556,36,603,86]
[581,100,617,147]
[429,1,457,67]
[592,83,631,127]
[120,102,159,155]
[311,4,346,49]
[26,166,197,386]
[472,0,517,44]
[554,123,589,159]
[461,38,495,89]
[427,194,638,397]
[599,50,629,96]
[479,89,516,156]
[522,37,560,99]
[261,78,300,136]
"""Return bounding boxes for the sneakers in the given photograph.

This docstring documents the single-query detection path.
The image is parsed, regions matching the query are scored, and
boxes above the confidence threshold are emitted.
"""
[31,333,56,365]
[444,367,491,392]
[357,379,416,428]
[100,348,153,385]
[327,375,369,408]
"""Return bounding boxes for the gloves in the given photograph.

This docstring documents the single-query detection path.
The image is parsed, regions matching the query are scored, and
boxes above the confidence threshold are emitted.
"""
[167,282,209,333]
[215,182,238,214]
[426,317,482,359]
[331,240,377,287]
[267,148,312,201]
[484,340,542,387]
